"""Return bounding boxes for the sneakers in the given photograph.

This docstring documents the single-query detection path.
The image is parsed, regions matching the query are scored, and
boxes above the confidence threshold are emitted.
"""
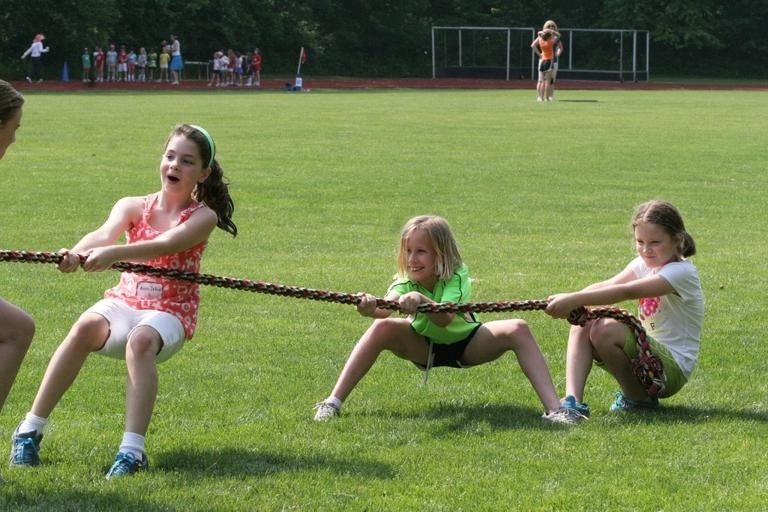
[312,400,340,422]
[608,392,658,412]
[540,408,578,426]
[37,79,43,84]
[104,451,150,481]
[560,394,590,419]
[9,422,44,468]
[26,77,32,84]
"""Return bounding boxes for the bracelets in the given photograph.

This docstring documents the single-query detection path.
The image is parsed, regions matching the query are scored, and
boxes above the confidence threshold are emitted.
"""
[550,31,553,34]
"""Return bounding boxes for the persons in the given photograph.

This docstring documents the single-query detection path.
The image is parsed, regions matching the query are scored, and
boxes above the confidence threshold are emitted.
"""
[538,28,561,103]
[21,35,50,83]
[0,78,36,411]
[531,20,564,103]
[78,30,262,88]
[312,213,588,426]
[546,202,704,421]
[10,125,237,479]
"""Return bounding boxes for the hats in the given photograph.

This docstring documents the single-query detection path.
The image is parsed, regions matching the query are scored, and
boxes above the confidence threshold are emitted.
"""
[33,34,45,42]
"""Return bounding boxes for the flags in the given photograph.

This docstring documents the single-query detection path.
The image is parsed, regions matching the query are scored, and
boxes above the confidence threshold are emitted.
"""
[301,46,307,65]
